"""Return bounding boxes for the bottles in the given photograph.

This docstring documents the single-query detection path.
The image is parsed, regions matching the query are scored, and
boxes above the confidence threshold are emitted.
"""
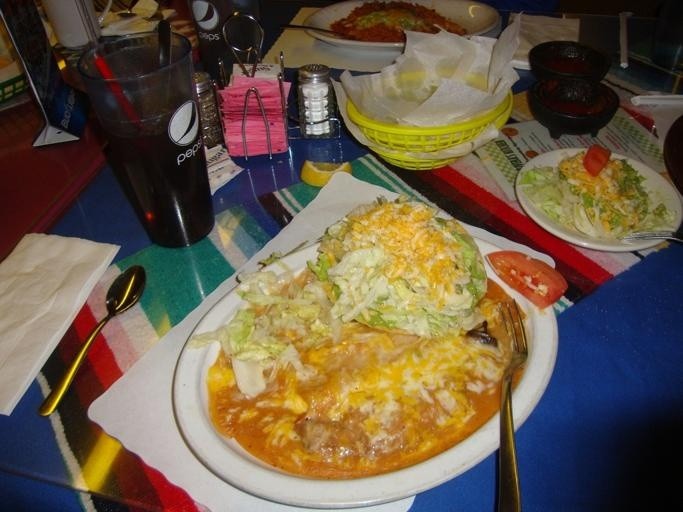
[189,72,220,146]
[296,63,334,140]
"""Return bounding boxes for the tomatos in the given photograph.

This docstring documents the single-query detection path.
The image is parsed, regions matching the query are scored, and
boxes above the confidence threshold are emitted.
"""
[487,251,569,309]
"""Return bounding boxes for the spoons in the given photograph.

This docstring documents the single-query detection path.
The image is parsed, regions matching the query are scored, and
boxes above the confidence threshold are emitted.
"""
[35,263,148,418]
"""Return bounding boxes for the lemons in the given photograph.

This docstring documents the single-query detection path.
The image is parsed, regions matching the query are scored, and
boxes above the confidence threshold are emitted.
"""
[301,160,351,187]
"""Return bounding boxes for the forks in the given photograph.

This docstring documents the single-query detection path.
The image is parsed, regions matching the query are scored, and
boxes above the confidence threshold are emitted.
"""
[491,301,532,511]
[626,230,682,246]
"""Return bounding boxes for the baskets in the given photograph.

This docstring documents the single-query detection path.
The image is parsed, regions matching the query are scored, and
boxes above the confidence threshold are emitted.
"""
[346,79,511,152]
[344,94,515,170]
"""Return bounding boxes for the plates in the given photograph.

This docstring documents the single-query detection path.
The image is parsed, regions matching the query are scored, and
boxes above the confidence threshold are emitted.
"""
[304,1,501,52]
[172,234,561,512]
[512,146,680,253]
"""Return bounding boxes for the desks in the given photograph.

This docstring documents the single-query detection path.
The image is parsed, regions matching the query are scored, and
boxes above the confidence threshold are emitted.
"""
[0,0,683,512]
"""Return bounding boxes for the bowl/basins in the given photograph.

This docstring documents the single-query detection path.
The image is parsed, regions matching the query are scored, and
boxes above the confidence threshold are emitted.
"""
[524,41,623,140]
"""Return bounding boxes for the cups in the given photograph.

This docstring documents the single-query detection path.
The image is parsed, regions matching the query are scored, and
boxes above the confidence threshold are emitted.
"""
[76,33,212,253]
